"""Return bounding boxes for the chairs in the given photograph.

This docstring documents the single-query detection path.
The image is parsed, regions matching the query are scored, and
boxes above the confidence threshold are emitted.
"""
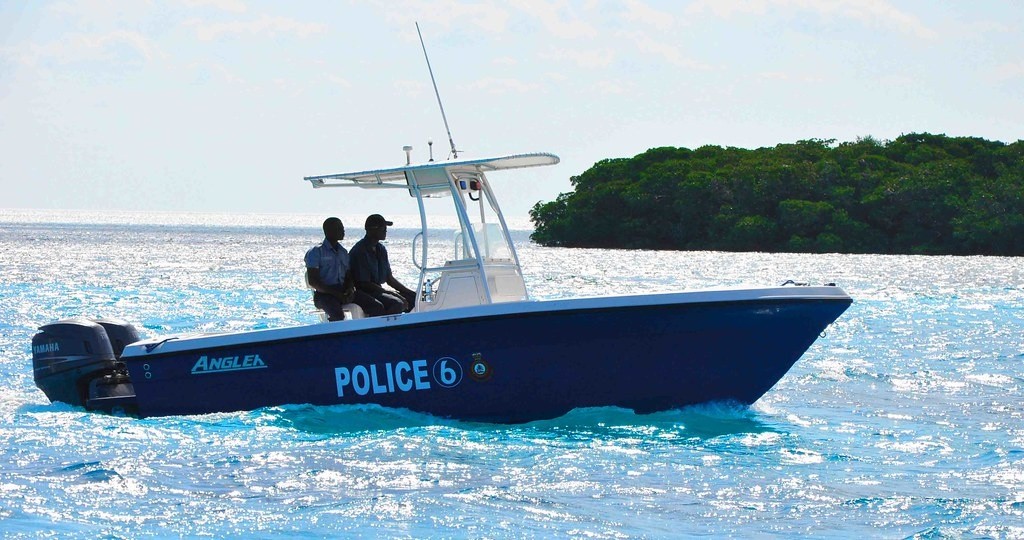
[305,271,369,324]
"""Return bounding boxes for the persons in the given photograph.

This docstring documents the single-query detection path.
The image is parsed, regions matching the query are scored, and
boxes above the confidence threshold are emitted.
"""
[303,217,384,322]
[349,213,416,316]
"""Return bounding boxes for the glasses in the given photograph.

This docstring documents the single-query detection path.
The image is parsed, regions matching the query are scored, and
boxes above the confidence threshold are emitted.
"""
[375,227,387,231]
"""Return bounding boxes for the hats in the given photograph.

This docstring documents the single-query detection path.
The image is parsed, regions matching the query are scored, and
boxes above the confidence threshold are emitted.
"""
[365,214,393,226]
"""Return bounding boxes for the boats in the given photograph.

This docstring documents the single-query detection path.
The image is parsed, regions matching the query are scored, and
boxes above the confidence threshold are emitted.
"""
[32,19,852,424]
